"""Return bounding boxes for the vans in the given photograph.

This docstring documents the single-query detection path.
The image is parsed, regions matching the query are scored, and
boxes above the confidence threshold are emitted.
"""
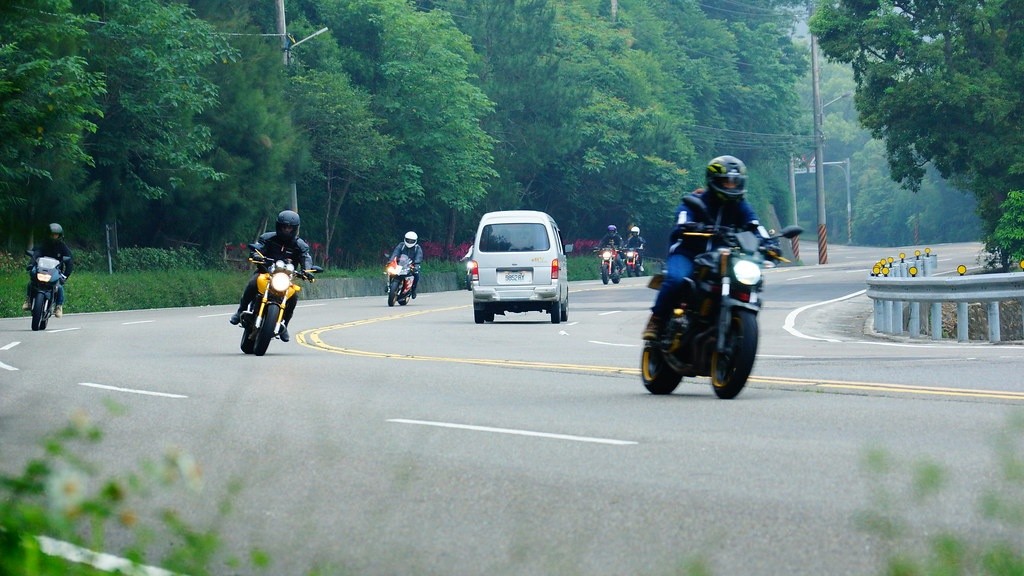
[466,208,575,324]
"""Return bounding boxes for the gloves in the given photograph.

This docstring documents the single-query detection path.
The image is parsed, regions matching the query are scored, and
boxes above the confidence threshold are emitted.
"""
[594,247,600,252]
[301,271,315,283]
[414,265,418,274]
[59,275,67,286]
[765,245,782,261]
[250,253,262,261]
[686,222,697,232]
[26,264,32,272]
[618,247,623,252]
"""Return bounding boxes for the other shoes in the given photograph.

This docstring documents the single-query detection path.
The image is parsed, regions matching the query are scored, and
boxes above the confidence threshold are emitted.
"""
[620,269,624,274]
[600,272,602,275]
[643,317,657,338]
[23,302,28,310]
[412,290,416,299]
[385,286,388,292]
[639,266,644,271]
[280,326,289,341]
[55,305,62,317]
[230,313,240,325]
[623,267,626,271]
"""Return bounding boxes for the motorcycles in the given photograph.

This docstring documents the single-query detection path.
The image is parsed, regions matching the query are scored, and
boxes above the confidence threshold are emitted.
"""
[593,239,624,285]
[383,252,421,306]
[621,243,645,278]
[639,204,805,401]
[236,242,324,356]
[24,249,71,331]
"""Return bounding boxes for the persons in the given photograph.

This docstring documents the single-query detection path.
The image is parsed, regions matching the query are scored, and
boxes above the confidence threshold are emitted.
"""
[642,155,782,340]
[22,223,74,318]
[459,238,475,262]
[384,231,423,299]
[627,226,646,271]
[229,210,313,342]
[594,225,624,274]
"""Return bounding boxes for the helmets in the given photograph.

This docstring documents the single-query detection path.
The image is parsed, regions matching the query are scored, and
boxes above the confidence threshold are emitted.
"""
[47,224,63,246]
[404,232,418,248]
[631,227,640,237]
[275,212,299,241]
[607,225,617,237]
[706,155,748,203]
